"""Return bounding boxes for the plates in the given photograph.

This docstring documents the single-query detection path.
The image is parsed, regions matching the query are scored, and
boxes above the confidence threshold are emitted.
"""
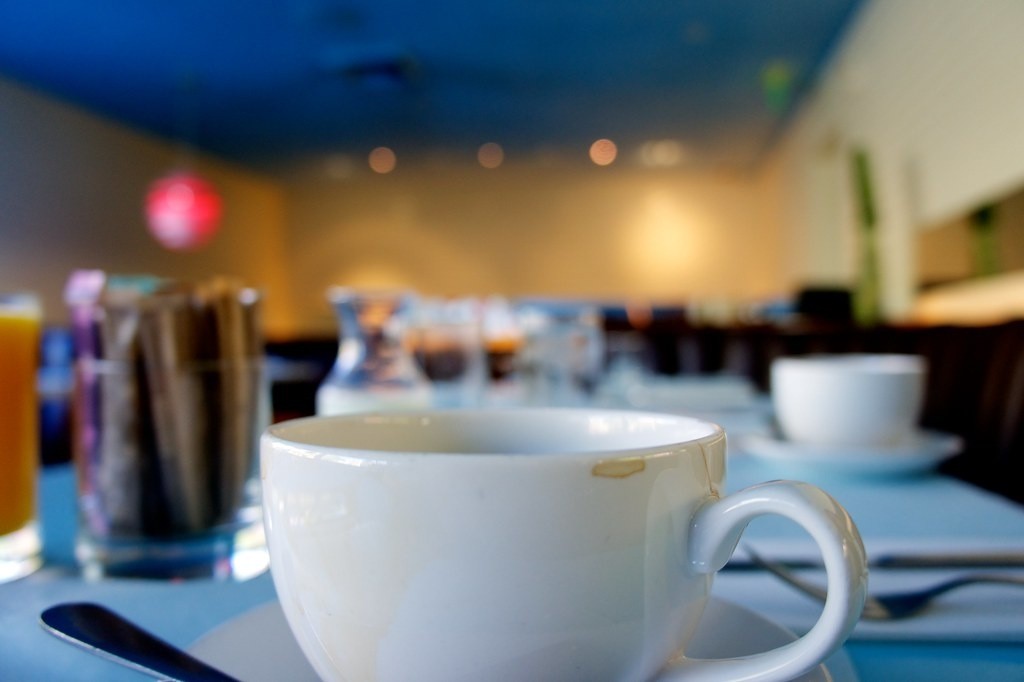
[743,426,963,483]
[161,592,834,682]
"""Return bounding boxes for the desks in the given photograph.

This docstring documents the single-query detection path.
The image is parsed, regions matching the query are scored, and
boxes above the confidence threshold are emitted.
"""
[0,373,1024,682]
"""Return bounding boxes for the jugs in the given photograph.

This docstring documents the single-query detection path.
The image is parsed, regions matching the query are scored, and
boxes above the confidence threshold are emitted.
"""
[316,285,433,417]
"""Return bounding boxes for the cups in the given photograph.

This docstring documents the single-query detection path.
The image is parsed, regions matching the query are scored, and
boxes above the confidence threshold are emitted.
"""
[0,296,45,585]
[771,354,926,453]
[259,408,869,682]
[79,281,252,579]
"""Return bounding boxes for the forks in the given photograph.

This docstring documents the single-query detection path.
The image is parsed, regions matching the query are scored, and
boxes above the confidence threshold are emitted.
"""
[746,537,1024,621]
